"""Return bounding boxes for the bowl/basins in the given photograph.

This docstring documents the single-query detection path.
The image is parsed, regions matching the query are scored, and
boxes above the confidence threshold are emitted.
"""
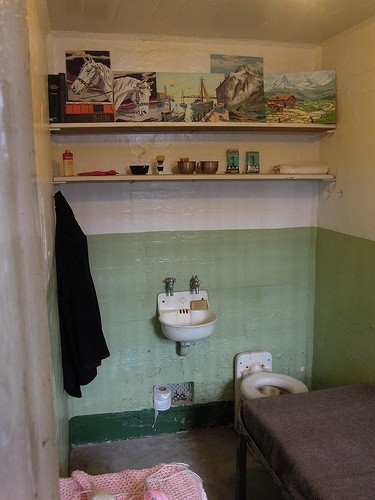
[129,165,150,175]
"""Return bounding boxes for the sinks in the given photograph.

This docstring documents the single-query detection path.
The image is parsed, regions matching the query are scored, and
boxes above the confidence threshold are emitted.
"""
[155,289,218,342]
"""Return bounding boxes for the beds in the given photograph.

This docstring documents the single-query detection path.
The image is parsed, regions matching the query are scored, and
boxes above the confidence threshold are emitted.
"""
[232,380,375,500]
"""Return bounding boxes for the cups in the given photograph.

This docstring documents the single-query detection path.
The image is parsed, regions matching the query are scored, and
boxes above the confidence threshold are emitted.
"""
[197,160,219,175]
[178,161,196,174]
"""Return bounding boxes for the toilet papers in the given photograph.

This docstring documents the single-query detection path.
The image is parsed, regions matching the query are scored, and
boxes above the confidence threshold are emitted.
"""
[152,384,173,411]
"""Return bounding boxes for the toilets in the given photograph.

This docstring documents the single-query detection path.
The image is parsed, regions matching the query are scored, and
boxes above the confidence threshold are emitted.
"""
[231,349,312,447]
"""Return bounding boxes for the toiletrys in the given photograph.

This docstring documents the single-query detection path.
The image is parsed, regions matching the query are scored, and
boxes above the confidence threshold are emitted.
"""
[62,145,76,177]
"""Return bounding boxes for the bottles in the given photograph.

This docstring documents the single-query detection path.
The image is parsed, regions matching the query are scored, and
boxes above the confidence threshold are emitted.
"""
[158,161,163,175]
[180,158,190,174]
[63,150,74,177]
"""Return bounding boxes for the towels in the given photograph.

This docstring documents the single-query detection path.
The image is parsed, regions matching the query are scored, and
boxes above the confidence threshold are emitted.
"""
[272,161,330,175]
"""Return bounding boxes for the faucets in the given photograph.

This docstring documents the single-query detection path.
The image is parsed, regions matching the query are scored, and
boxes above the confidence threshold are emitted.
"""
[189,273,202,294]
[165,275,177,297]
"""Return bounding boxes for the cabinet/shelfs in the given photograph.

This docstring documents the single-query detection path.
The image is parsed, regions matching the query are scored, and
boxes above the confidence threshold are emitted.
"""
[49,120,337,184]
